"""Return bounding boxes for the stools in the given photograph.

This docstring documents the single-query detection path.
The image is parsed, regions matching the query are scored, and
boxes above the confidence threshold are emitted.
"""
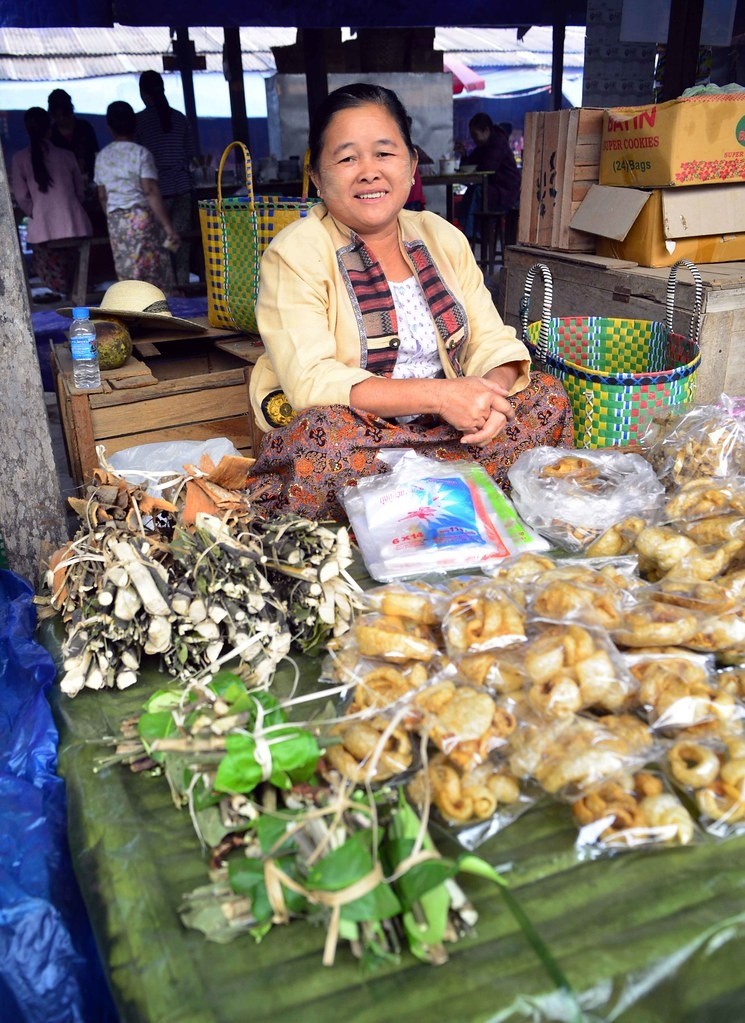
[469,212,506,276]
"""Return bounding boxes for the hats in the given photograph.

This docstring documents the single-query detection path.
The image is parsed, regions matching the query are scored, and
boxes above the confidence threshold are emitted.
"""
[55,279,208,332]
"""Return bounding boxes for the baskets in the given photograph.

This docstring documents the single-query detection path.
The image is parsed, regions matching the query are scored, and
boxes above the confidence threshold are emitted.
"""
[198,141,324,336]
[518,259,704,449]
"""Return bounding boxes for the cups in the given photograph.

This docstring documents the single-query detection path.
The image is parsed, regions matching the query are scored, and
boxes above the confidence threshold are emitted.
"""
[440,159,454,175]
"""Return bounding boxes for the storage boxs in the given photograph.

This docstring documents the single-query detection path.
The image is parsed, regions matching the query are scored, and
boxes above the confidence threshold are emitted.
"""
[598,93,745,189]
[567,184,745,269]
[517,108,604,253]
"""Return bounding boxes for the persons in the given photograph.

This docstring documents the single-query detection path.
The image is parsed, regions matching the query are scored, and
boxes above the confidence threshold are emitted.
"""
[248,84,575,521]
[11,70,205,300]
[461,112,524,242]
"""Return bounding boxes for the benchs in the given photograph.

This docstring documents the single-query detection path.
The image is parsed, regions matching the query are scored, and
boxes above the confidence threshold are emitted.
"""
[43,229,206,307]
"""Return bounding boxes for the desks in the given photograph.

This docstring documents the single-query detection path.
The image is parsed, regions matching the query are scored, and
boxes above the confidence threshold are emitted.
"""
[9,171,496,278]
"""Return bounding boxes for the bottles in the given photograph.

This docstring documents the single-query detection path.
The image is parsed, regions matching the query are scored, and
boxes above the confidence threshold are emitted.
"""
[70,307,101,389]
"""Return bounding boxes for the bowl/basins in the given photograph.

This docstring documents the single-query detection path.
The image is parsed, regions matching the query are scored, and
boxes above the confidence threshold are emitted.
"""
[454,165,478,173]
